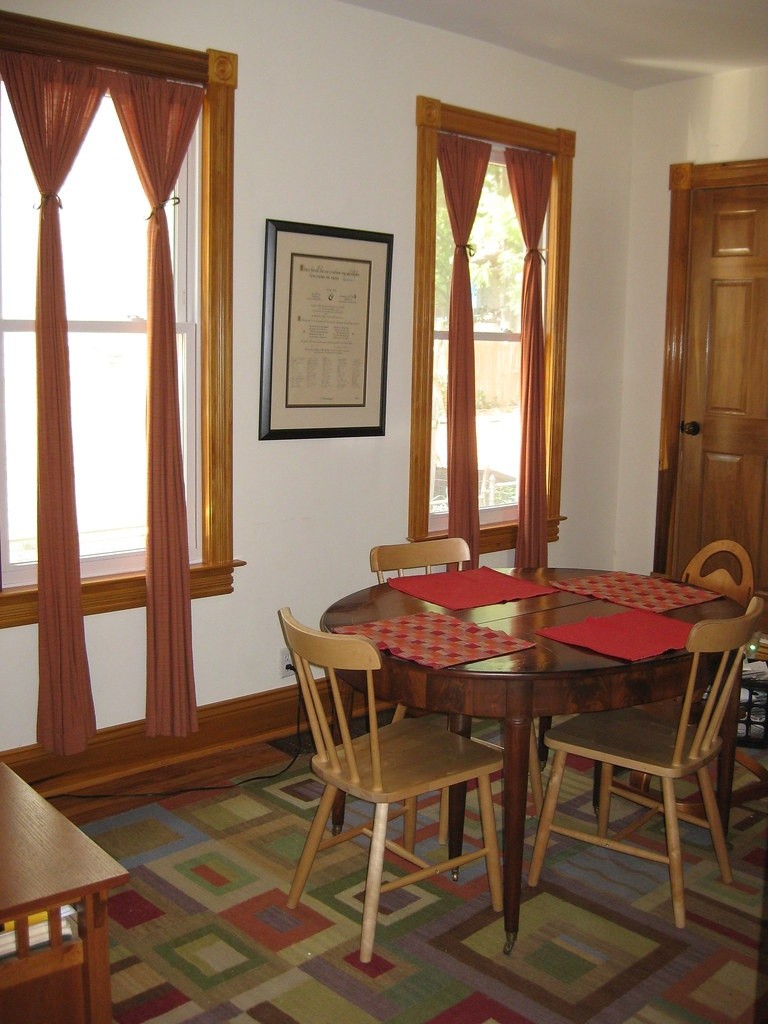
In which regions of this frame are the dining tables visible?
[318,567,745,953]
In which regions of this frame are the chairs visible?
[528,592,764,928]
[277,607,504,962]
[368,537,542,846]
[628,538,768,795]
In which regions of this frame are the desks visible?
[0,757,131,1024]
